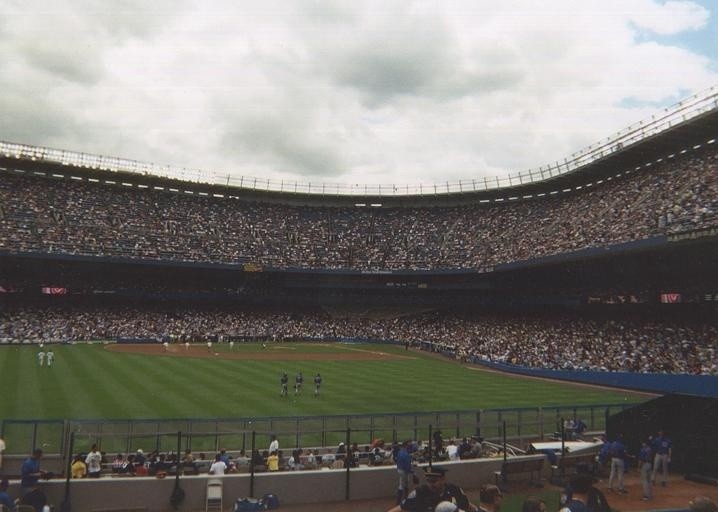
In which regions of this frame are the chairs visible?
[204,479,223,512]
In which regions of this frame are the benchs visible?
[491,459,547,485]
[550,451,601,484]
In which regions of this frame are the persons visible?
[280,372,288,398]
[262,343,266,349]
[47,349,55,367]
[574,416,587,437]
[1,171,410,338]
[562,416,573,437]
[161,342,168,351]
[412,146,716,375]
[687,494,717,510]
[403,340,410,350]
[37,350,46,366]
[314,372,321,396]
[185,342,191,350]
[228,341,233,351]
[294,371,304,397]
[1,428,675,509]
[207,340,213,352]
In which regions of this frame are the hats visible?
[422,466,448,480]
